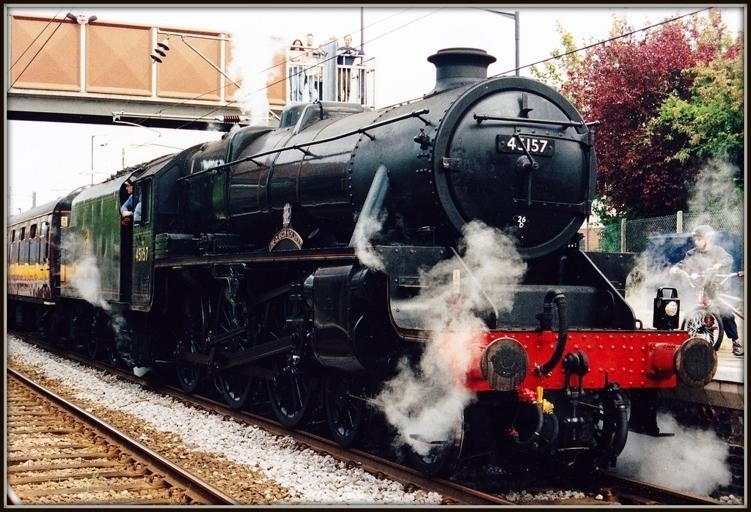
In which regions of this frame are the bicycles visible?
[676,266,745,352]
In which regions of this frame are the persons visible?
[120,176,137,218]
[678,224,744,357]
[289,32,365,103]
[132,187,142,223]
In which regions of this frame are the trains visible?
[7,44,719,496]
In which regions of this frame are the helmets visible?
[693,225,714,239]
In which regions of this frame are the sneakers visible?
[733,339,743,356]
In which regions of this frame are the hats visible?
[125,176,136,185]
